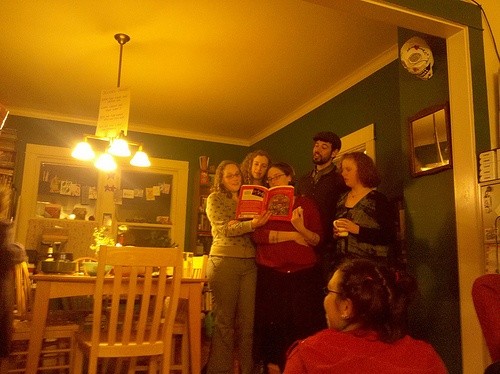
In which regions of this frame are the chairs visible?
[72,245,183,374]
[13,253,207,374]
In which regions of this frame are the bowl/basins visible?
[42,260,79,274]
[80,262,113,277]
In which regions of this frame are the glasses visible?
[321,286,343,296]
[266,174,286,183]
[222,172,241,179]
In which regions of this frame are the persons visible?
[240,150,272,184]
[283,258,448,374]
[297,131,341,280]
[332,152,397,261]
[250,161,323,374]
[206,161,271,374]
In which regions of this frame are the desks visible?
[26,275,208,374]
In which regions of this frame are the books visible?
[235,184,294,220]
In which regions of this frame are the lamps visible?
[70,33,152,171]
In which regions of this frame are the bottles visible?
[115,234,126,247]
[45,245,55,261]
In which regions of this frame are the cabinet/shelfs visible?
[191,169,217,256]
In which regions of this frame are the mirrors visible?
[407,102,454,178]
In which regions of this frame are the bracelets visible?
[272,230,278,243]
[305,232,316,245]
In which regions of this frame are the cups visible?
[333,220,349,237]
[182,252,194,279]
